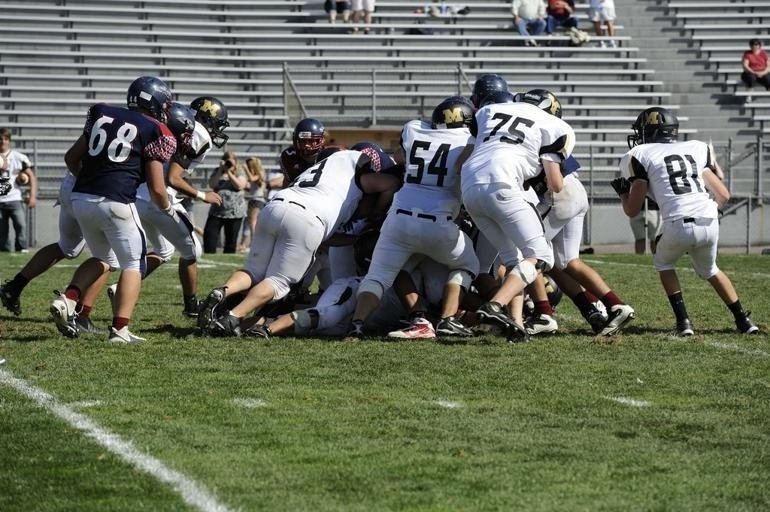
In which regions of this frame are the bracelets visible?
[161,201,172,215]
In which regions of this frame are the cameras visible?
[224,160,231,168]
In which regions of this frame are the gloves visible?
[610,176,630,195]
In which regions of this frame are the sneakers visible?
[387,294,558,345]
[0,280,22,316]
[342,319,368,339]
[182,286,272,338]
[341,217,374,236]
[582,299,636,336]
[676,318,694,336]
[50,283,147,344]
[734,310,759,335]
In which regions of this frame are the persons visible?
[0,103,195,337]
[589,0,617,48]
[510,0,547,46]
[324,0,352,34]
[351,0,375,34]
[611,108,759,334]
[741,39,770,103]
[50,77,180,344]
[108,97,229,316]
[0,128,36,253]
[546,0,579,46]
[619,196,662,255]
[196,74,634,340]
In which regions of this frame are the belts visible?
[274,198,324,225]
[396,209,452,222]
[683,218,695,223]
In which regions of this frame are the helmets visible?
[127,76,231,151]
[293,118,328,163]
[627,107,679,150]
[543,273,563,312]
[431,74,562,129]
